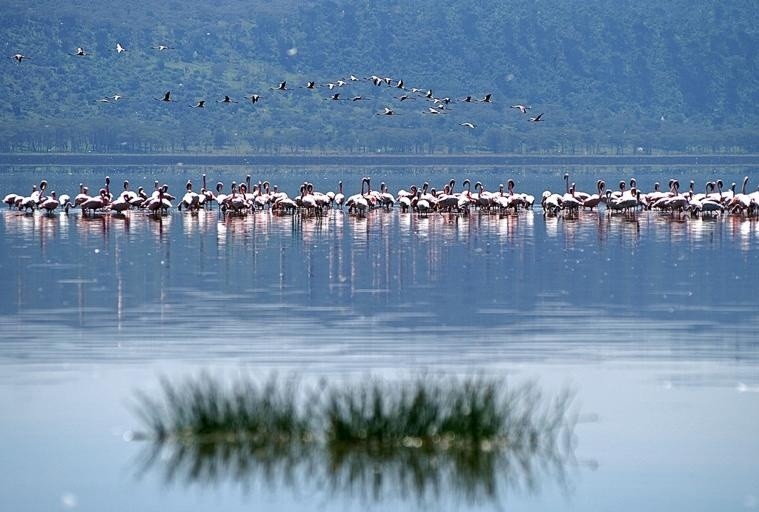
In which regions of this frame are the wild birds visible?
[108,43,127,54]
[510,103,532,113]
[150,46,175,52]
[456,94,494,104]
[397,178,535,215]
[365,75,410,91]
[458,122,478,129]
[380,94,413,116]
[94,99,114,104]
[527,113,545,122]
[68,48,94,58]
[6,175,176,214]
[325,93,371,102]
[189,94,265,108]
[269,76,363,91]
[104,95,122,102]
[6,54,32,62]
[412,87,453,115]
[153,91,180,103]
[540,174,759,218]
[346,178,394,214]
[177,174,344,214]
